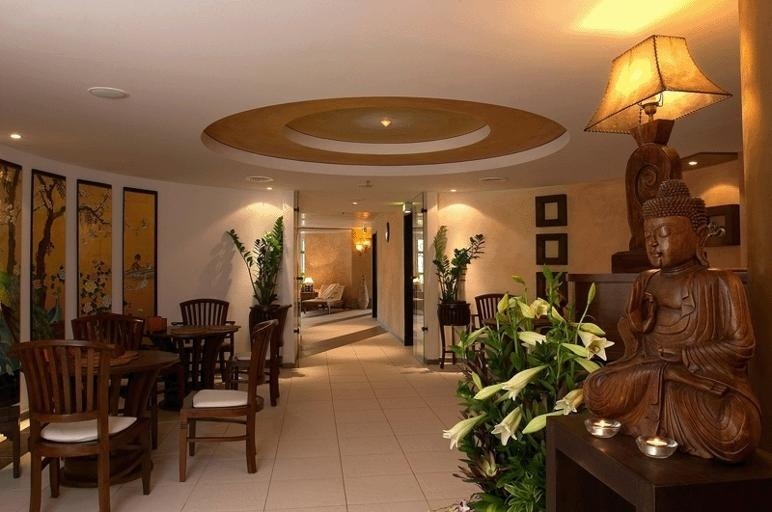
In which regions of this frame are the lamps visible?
[584,35,732,273]
[303,277,313,291]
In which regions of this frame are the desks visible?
[10,342,179,489]
[568,271,748,361]
[546,415,772,512]
[300,292,318,312]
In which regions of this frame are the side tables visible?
[439,323,470,369]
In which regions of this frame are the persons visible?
[583,178,762,464]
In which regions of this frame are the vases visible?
[358,272,369,309]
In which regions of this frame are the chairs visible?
[16,337,154,512]
[302,284,344,314]
[177,318,276,483]
[475,293,516,328]
[147,299,280,411]
[69,318,146,352]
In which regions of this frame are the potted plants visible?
[225,216,292,345]
[432,225,485,325]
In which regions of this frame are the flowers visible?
[442,265,615,512]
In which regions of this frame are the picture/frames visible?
[30,168,67,341]
[76,178,113,318]
[0,159,23,374]
[123,186,158,317]
